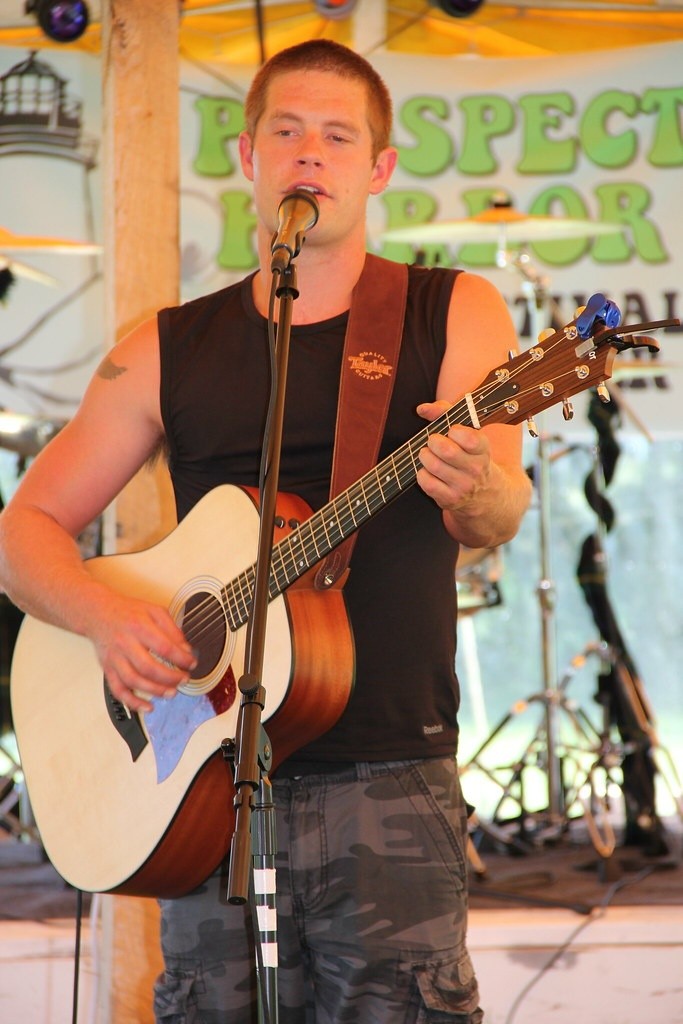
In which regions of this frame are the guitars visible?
[8,294,682,901]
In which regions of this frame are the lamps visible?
[24,0,88,42]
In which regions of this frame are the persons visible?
[0,38,532,1024]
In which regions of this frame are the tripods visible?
[457,434,683,881]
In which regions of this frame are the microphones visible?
[270,189,321,275]
